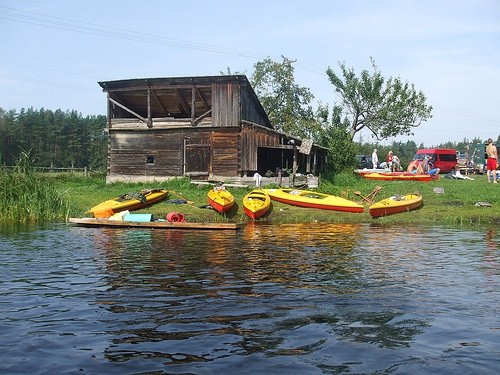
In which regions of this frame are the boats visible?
[368,193,423,217]
[207,181,235,214]
[68,217,246,230]
[85,188,170,217]
[352,167,433,182]
[242,188,273,219]
[262,188,370,214]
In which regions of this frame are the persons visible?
[387,151,400,172]
[484,137,499,184]
[372,149,378,170]
[252,170,263,188]
[450,165,477,181]
[408,156,440,176]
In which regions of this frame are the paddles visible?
[167,189,195,206]
[354,186,382,204]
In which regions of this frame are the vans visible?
[408,147,458,173]
